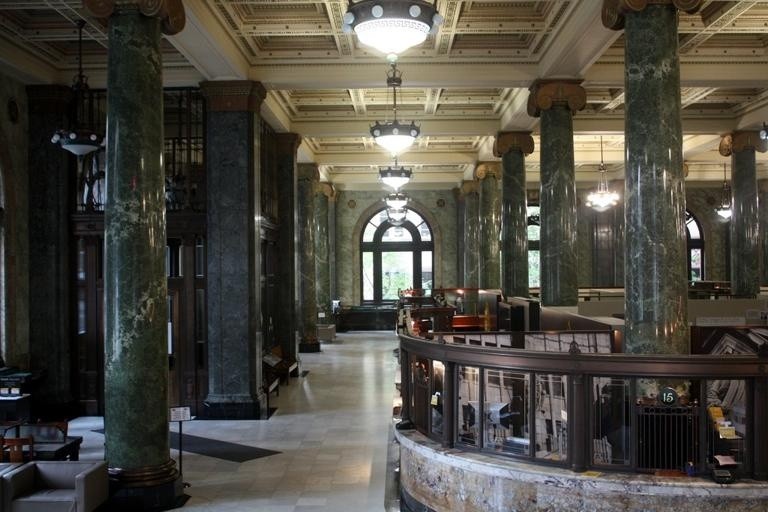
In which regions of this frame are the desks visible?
[0,387,32,420]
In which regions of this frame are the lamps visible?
[381,192,408,228]
[379,162,413,196]
[49,19,103,158]
[341,0,441,62]
[721,163,732,210]
[585,135,621,213]
[366,63,422,160]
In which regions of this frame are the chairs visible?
[0,420,83,460]
[1,462,107,512]
